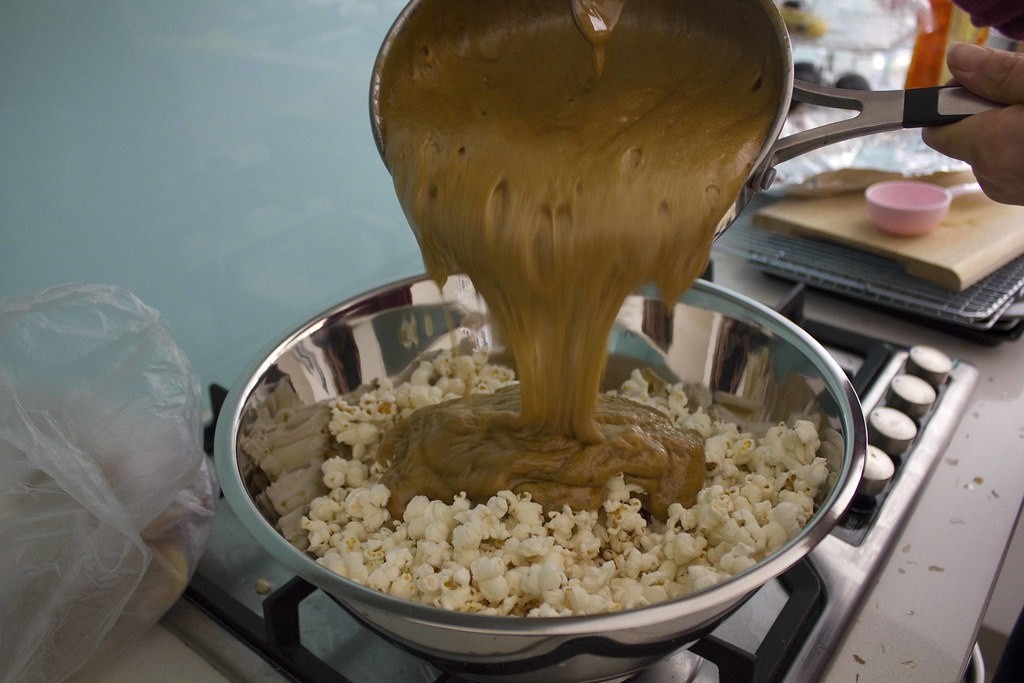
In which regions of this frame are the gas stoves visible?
[165,273,978,683]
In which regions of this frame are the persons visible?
[921,0,1024,207]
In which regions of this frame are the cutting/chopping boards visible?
[751,183,1024,290]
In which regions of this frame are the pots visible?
[372,1,1004,177]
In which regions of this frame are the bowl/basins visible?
[212,265,867,683]
[867,181,953,237]
